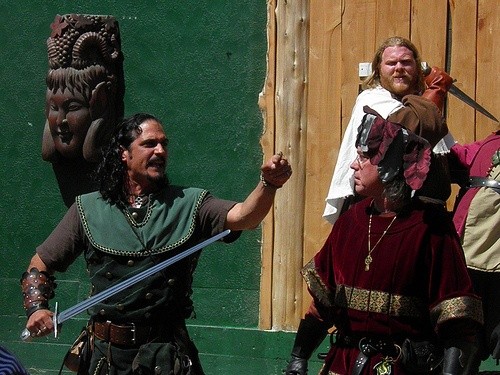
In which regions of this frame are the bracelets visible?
[260,173,282,190]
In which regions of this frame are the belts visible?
[335,330,431,357]
[88,321,171,346]
[465,178,500,189]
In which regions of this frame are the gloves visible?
[286,319,327,375]
[419,66,453,107]
[442,344,481,375]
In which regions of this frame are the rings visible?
[41,327,47,333]
[37,330,42,336]
[286,171,290,176]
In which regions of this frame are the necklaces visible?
[126,184,155,206]
[365,199,397,271]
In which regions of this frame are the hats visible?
[356,105,431,190]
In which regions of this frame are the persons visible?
[322,36,458,225]
[441,130,500,375]
[42,13,125,162]
[286,104,484,375]
[22,114,292,375]
